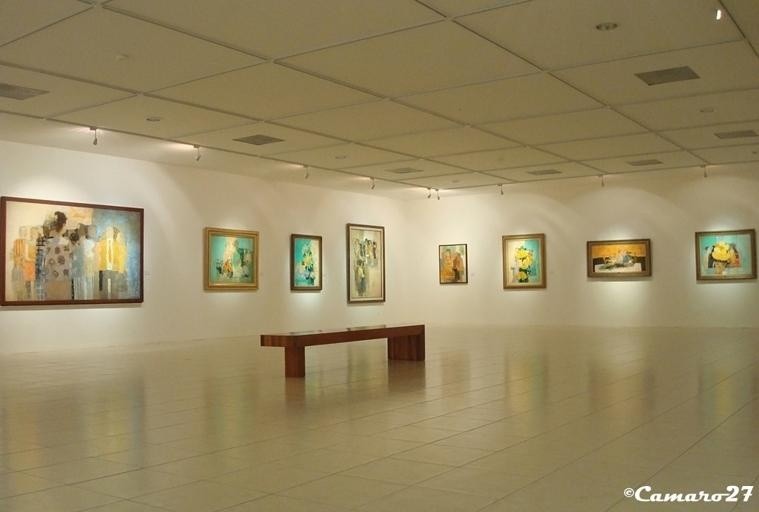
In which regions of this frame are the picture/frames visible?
[500,229,757,290]
[438,243,469,285]
[202,223,387,305]
[1,195,146,306]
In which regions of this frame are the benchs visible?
[259,321,427,378]
